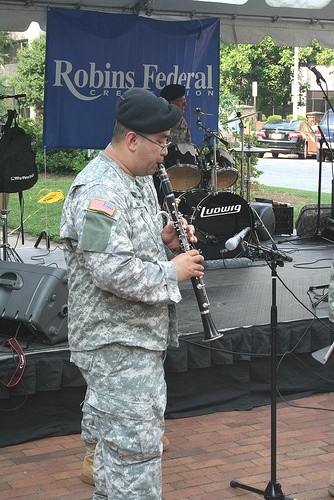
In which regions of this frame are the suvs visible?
[314,108,334,163]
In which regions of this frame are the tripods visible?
[277,140,333,244]
[0,94,26,263]
[230,236,297,500]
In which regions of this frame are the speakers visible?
[0,259,69,346]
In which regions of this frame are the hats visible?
[115,87,182,133]
[160,84,185,101]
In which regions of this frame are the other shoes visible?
[162,437,169,451]
[81,456,94,486]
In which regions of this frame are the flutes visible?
[158,163,225,343]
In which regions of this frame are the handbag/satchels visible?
[0,109,38,193]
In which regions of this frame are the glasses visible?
[125,130,172,152]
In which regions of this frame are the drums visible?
[200,146,238,189]
[176,189,253,260]
[163,142,202,189]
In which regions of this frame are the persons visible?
[59,88,205,500]
[160,84,191,145]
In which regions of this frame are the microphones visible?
[308,65,326,83]
[225,227,252,251]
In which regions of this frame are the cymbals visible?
[223,112,256,124]
[229,147,271,153]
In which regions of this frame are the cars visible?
[256,119,316,159]
